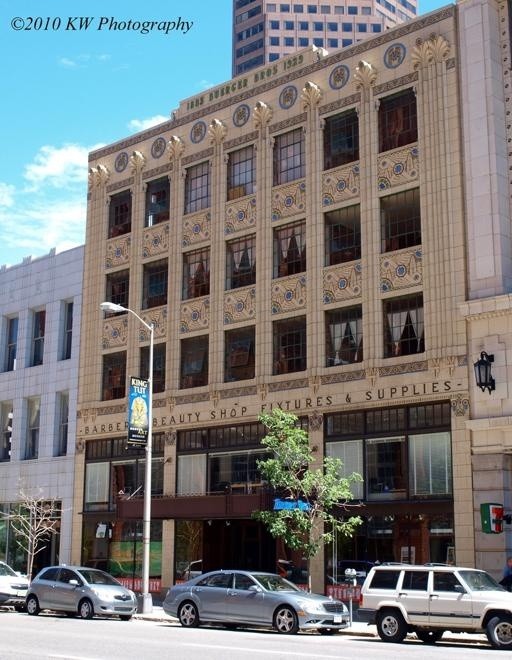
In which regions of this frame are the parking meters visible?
[344,568,357,626]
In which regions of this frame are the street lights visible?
[100,302,154,614]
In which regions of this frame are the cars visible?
[84,558,142,579]
[327,560,374,582]
[0,560,138,621]
[164,569,350,633]
[182,560,203,580]
[282,570,338,585]
[358,562,512,649]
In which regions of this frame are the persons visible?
[502,556,511,577]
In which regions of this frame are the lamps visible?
[473,352,494,394]
